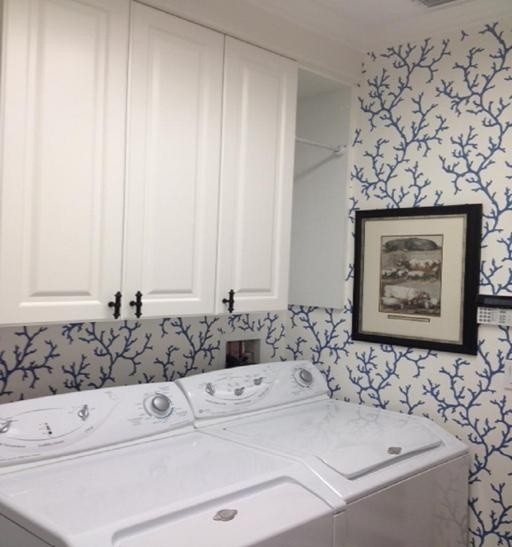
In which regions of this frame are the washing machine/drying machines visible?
[0,381,347,547]
[176,360,470,546]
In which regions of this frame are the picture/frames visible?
[351,203,483,356]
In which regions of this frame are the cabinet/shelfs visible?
[214,33,299,315]
[0,0,225,327]
[288,62,354,310]
